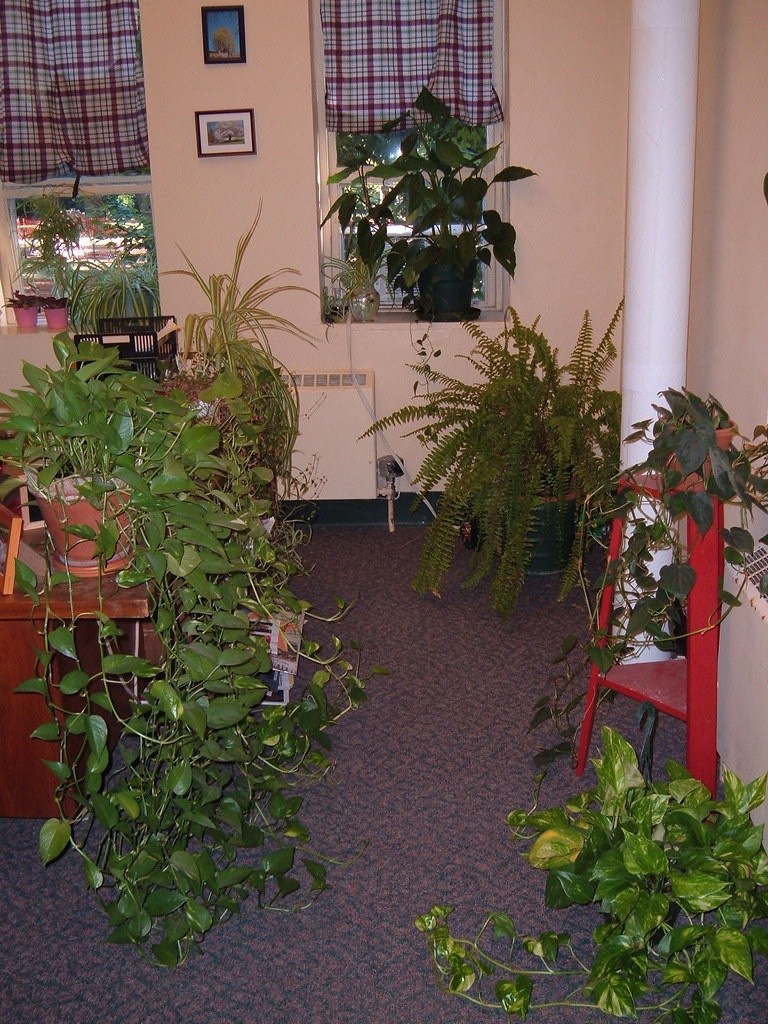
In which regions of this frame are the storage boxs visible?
[72,315,178,382]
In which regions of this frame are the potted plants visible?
[0,84,768,1024]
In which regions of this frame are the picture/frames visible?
[194,108,257,158]
[201,5,246,64]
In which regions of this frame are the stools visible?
[575,476,724,800]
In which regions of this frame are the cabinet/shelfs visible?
[0,528,163,819]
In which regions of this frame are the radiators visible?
[273,368,378,501]
